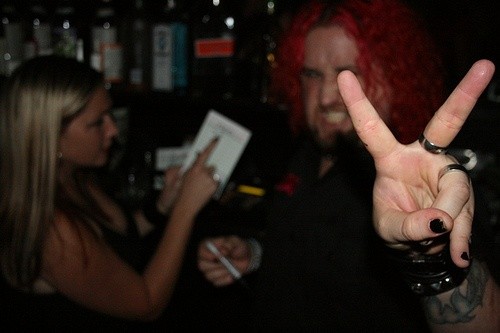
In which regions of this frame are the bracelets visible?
[143,204,169,226]
[403,244,471,296]
[248,236,261,273]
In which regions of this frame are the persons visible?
[0,55,220,333]
[337,60,500,333]
[197,0,500,333]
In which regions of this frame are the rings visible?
[439,164,471,182]
[419,132,449,155]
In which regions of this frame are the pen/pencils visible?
[205,243,242,279]
